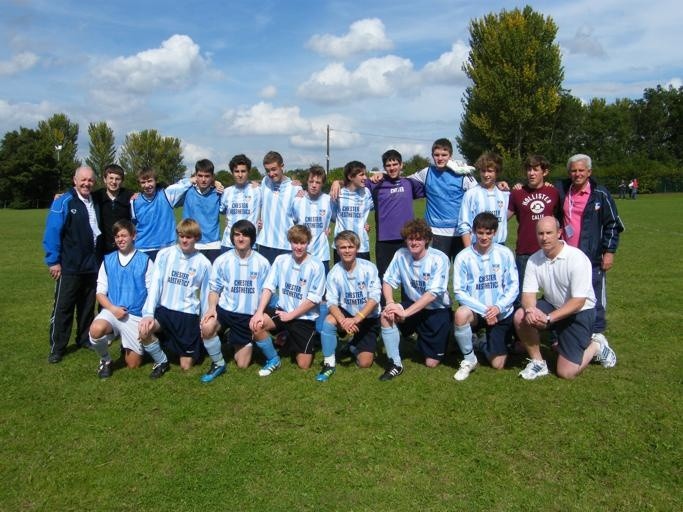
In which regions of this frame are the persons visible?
[43,137,626,364]
[512,217,616,380]
[374,218,451,381]
[630,178,638,200]
[86,219,154,378]
[247,224,326,377]
[198,218,271,383]
[315,229,384,381]
[627,179,634,199]
[451,210,521,379]
[136,217,214,379]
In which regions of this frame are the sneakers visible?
[85,344,94,352]
[519,358,546,380]
[200,362,226,380]
[592,333,616,368]
[97,360,113,378]
[49,354,62,363]
[473,334,478,345]
[454,357,478,381]
[108,333,117,346]
[315,362,336,380]
[476,334,487,350]
[150,359,170,379]
[258,359,282,377]
[275,330,288,346]
[381,363,403,380]
